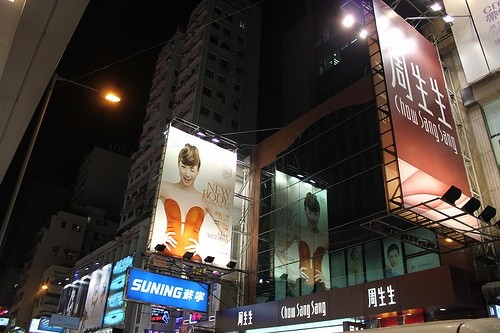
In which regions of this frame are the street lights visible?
[0,72,121,248]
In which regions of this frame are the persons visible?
[66,289,76,317]
[157,142,232,264]
[275,192,330,288]
[350,246,360,286]
[385,243,403,278]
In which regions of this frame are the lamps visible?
[440,184,500,230]
